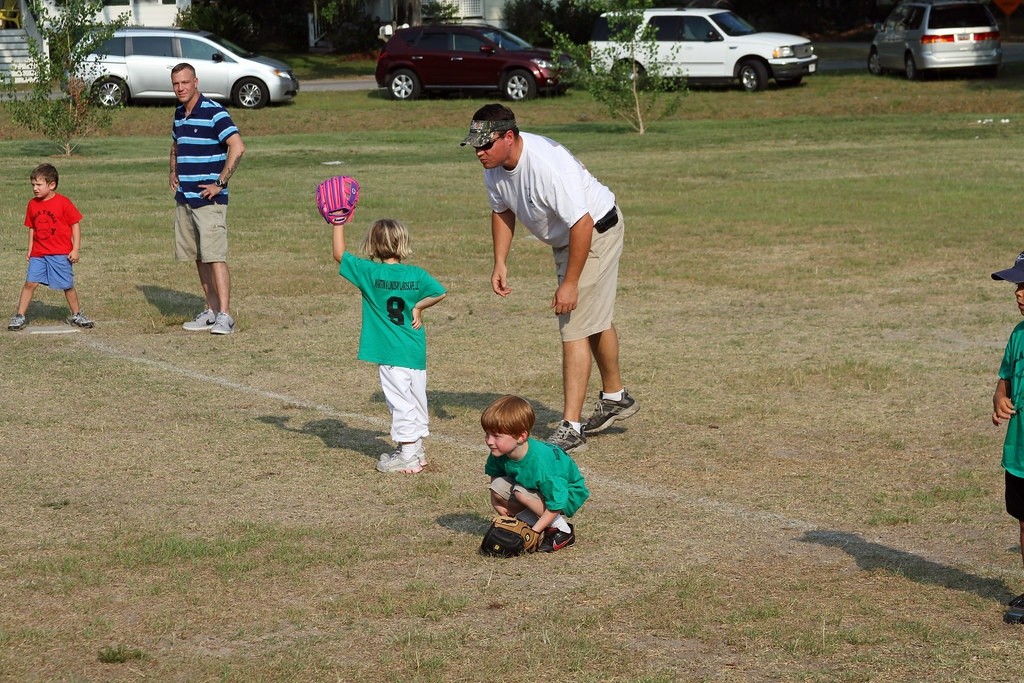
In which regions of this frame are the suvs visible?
[867,0,1004,81]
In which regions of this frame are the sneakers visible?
[376,445,428,474]
[182,309,216,330]
[536,523,575,551]
[584,387,640,432]
[7,313,26,331]
[210,311,235,335]
[66,312,94,328]
[542,420,589,454]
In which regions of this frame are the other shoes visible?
[1003,593,1024,624]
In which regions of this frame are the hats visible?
[460,120,516,146]
[991,250,1024,283]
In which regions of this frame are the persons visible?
[480,394,590,552]
[315,176,448,474]
[987,252,1024,623]
[8,163,95,330]
[169,63,245,335]
[461,104,640,450]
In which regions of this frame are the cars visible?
[586,7,818,93]
[58,25,300,109]
[375,23,581,101]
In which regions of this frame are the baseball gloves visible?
[480,516,545,559]
[315,175,361,225]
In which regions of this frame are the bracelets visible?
[170,171,176,173]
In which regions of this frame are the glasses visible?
[474,130,509,150]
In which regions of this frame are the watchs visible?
[215,178,228,189]
